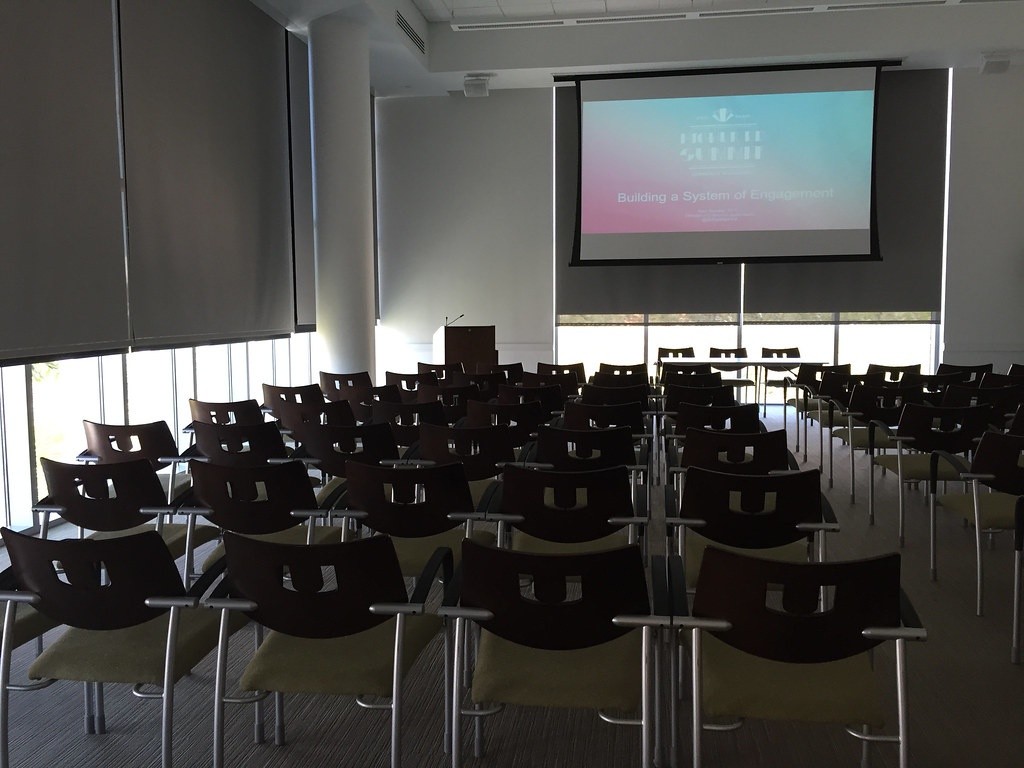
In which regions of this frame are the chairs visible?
[670,544,929,768]
[204,529,469,768]
[1,526,266,768]
[1,346,1024,682]
[438,537,668,768]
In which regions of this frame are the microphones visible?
[445,313,464,326]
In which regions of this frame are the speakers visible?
[463,80,489,97]
[979,56,1011,74]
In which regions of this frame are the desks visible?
[659,356,830,417]
